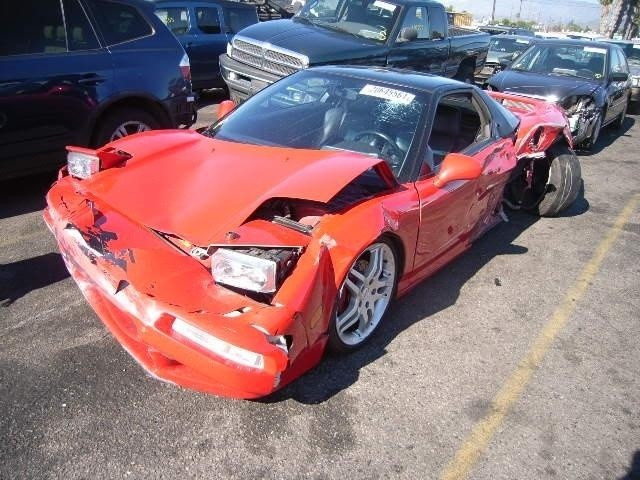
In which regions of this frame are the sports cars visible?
[30,59,589,404]
